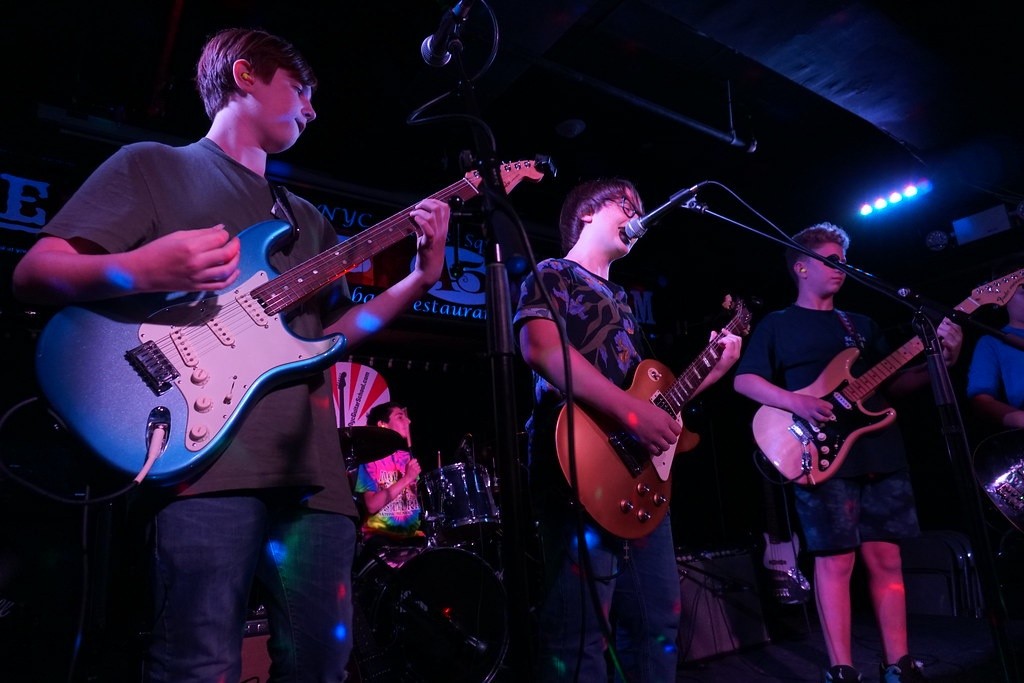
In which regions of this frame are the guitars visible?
[972,426,1024,535]
[547,293,754,541]
[761,484,812,606]
[31,157,559,494]
[751,268,1024,488]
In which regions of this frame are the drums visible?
[416,459,503,545]
[350,544,512,683]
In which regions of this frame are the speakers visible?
[674,547,772,663]
[238,617,272,683]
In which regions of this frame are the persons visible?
[515,177,742,683]
[731,221,963,683]
[967,262,1024,429]
[355,403,421,537]
[15,30,450,683]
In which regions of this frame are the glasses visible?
[601,195,643,221]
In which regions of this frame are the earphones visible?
[800,268,806,273]
[242,73,254,85]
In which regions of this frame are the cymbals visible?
[337,425,408,472]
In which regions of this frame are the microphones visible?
[421,0,473,68]
[625,180,708,239]
[464,639,488,651]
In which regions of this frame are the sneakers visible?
[879,654,928,683]
[824,663,863,683]
